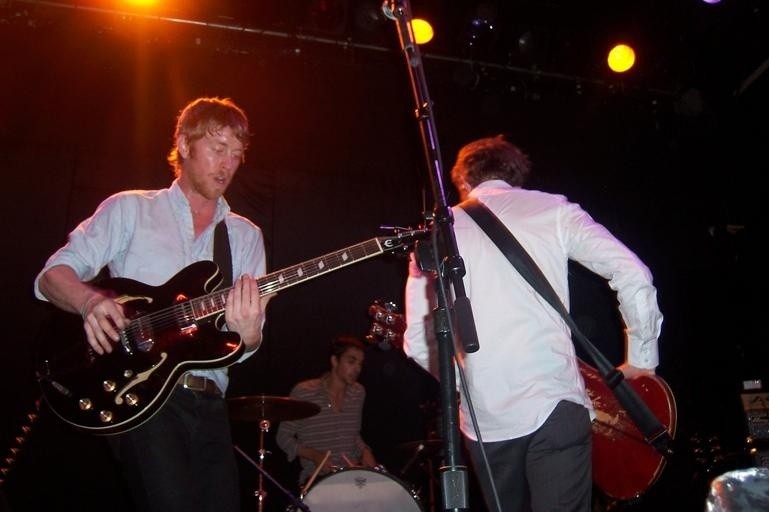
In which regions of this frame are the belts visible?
[176,373,222,397]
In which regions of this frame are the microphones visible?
[382,0,400,19]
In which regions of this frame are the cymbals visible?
[390,438,449,459]
[226,396,319,421]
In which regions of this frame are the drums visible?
[287,465,425,511]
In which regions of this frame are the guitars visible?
[35,226,430,437]
[363,300,677,500]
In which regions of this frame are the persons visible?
[403,138,663,511]
[276,336,376,490]
[34,97,276,398]
